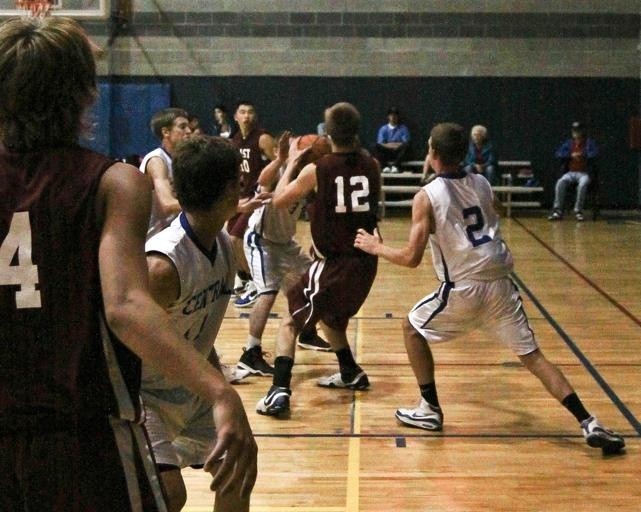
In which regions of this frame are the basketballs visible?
[299,135,332,162]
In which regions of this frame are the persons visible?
[352,122,626,450]
[373,103,413,172]
[547,120,601,220]
[461,124,498,186]
[0,12,258,511]
[316,102,336,135]
[213,103,240,138]
[139,106,194,242]
[235,127,334,377]
[189,115,204,136]
[224,99,278,308]
[138,134,251,512]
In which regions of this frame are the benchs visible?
[378,159,545,218]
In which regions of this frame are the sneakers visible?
[221,326,370,415]
[233,280,261,308]
[391,162,400,173]
[395,396,443,431]
[548,209,563,220]
[574,212,585,221]
[580,414,625,449]
[382,164,391,172]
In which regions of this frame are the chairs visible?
[551,156,600,222]
